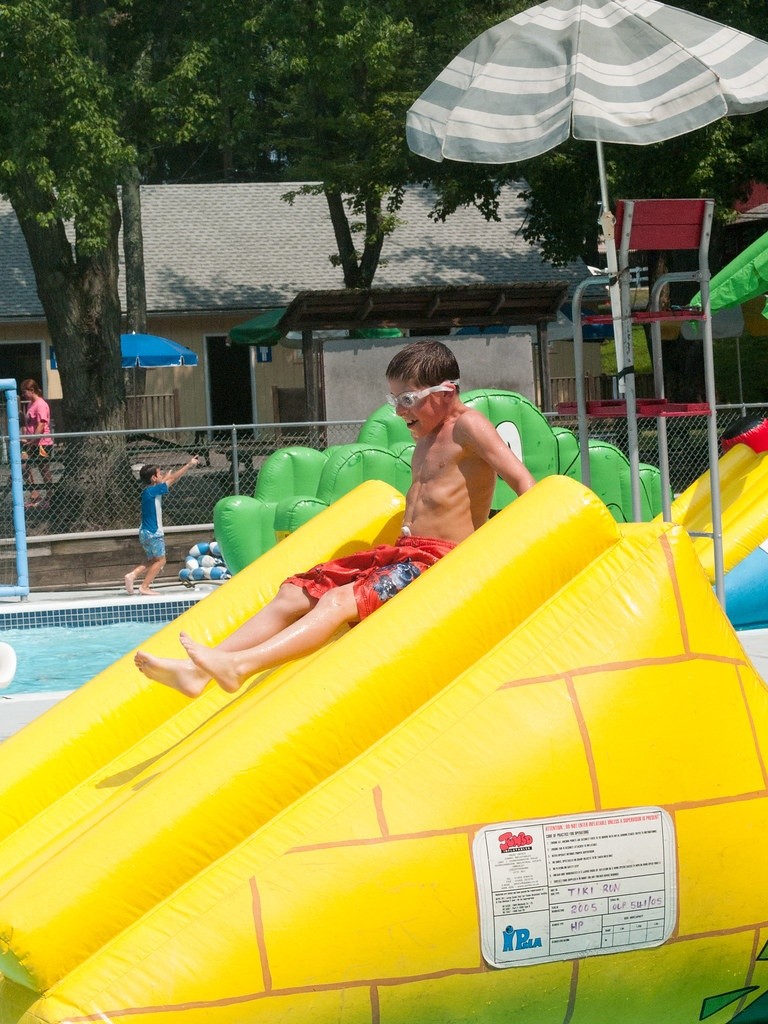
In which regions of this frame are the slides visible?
[0,388,768,1024]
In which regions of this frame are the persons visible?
[18,379,54,501]
[125,455,200,595]
[133,340,535,698]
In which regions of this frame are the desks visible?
[0,456,66,506]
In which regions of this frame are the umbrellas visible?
[120,334,199,366]
[405,0,768,393]
[229,308,404,348]
[690,231,768,320]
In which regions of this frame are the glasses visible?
[385,385,454,408]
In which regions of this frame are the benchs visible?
[127,445,213,467]
[0,483,61,491]
[215,446,277,472]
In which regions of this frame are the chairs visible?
[573,197,725,610]
